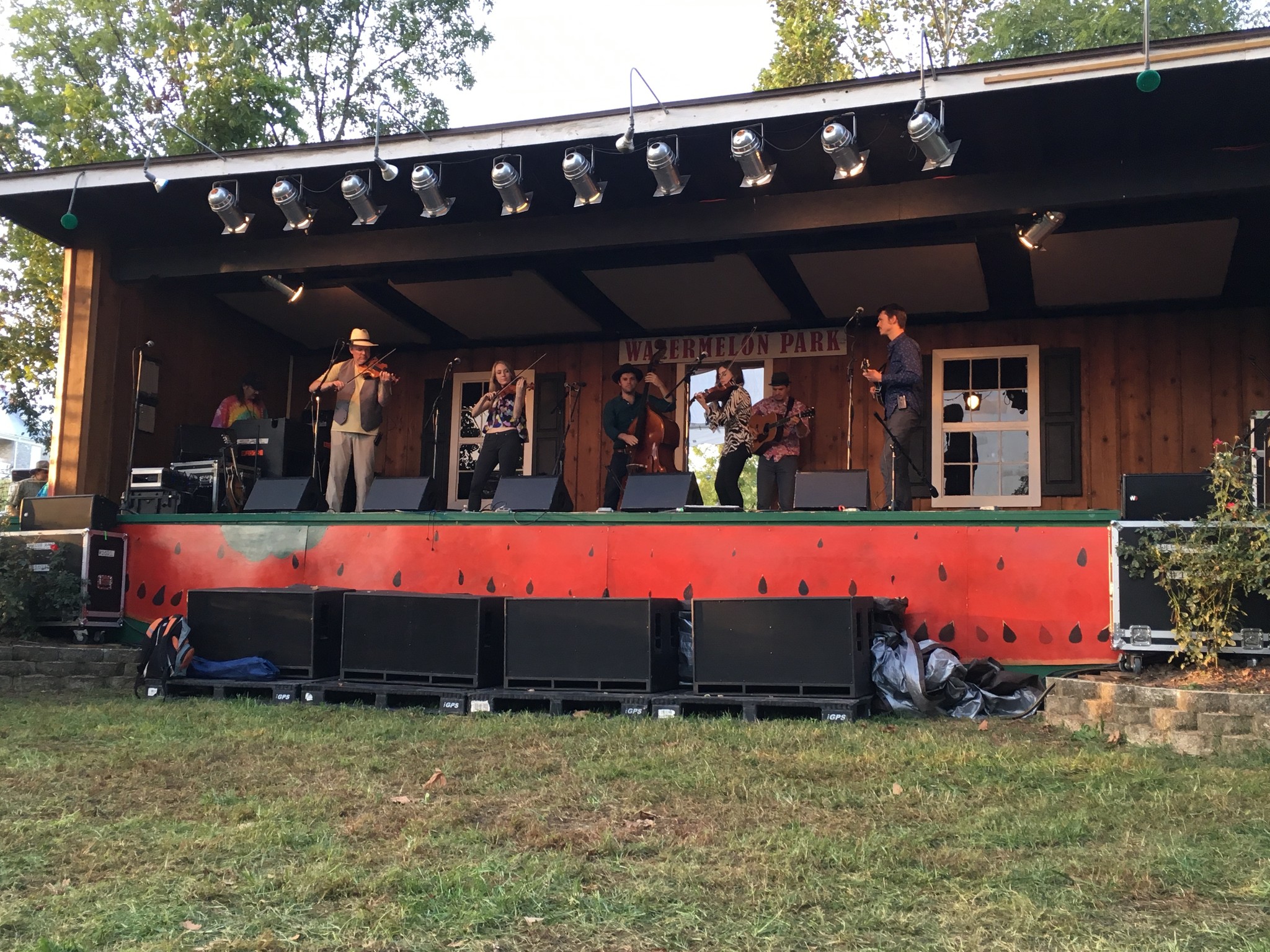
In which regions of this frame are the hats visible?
[342,328,378,346]
[768,371,791,386]
[29,460,49,474]
[611,363,643,385]
[248,369,268,389]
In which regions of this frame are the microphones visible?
[134,341,155,349]
[695,352,709,361]
[339,337,353,345]
[855,307,864,315]
[573,382,587,387]
[928,484,939,498]
[449,358,461,366]
[564,383,578,392]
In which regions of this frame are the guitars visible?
[222,433,248,513]
[749,407,815,457]
[861,358,885,407]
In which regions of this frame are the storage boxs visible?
[170,460,261,513]
[1110,520,1270,654]
[0,527,129,627]
[1242,410,1270,519]
[188,586,876,699]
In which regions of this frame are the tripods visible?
[119,348,144,516]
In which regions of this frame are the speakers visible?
[1120,473,1250,521]
[243,476,329,513]
[362,475,448,511]
[174,423,239,463]
[793,470,872,510]
[187,587,895,697]
[490,475,575,513]
[620,471,704,513]
[19,494,118,531]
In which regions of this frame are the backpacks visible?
[137,613,194,680]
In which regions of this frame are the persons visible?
[749,372,812,511]
[602,363,676,511]
[4,460,50,516]
[309,328,393,512]
[694,360,754,512]
[211,373,268,428]
[468,360,526,512]
[863,303,924,511]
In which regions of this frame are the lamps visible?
[374,101,431,181]
[962,391,982,411]
[561,143,608,208]
[729,122,777,189]
[907,28,962,173]
[339,169,388,225]
[411,161,456,219]
[207,179,256,235]
[820,109,872,181]
[271,174,318,235]
[259,272,305,303]
[143,121,227,193]
[1014,210,1066,251]
[492,154,534,216]
[646,133,691,197]
[615,67,669,154]
[1003,388,1027,414]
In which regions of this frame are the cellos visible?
[621,339,682,490]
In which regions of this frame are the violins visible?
[689,381,739,406]
[499,377,535,398]
[358,357,400,385]
